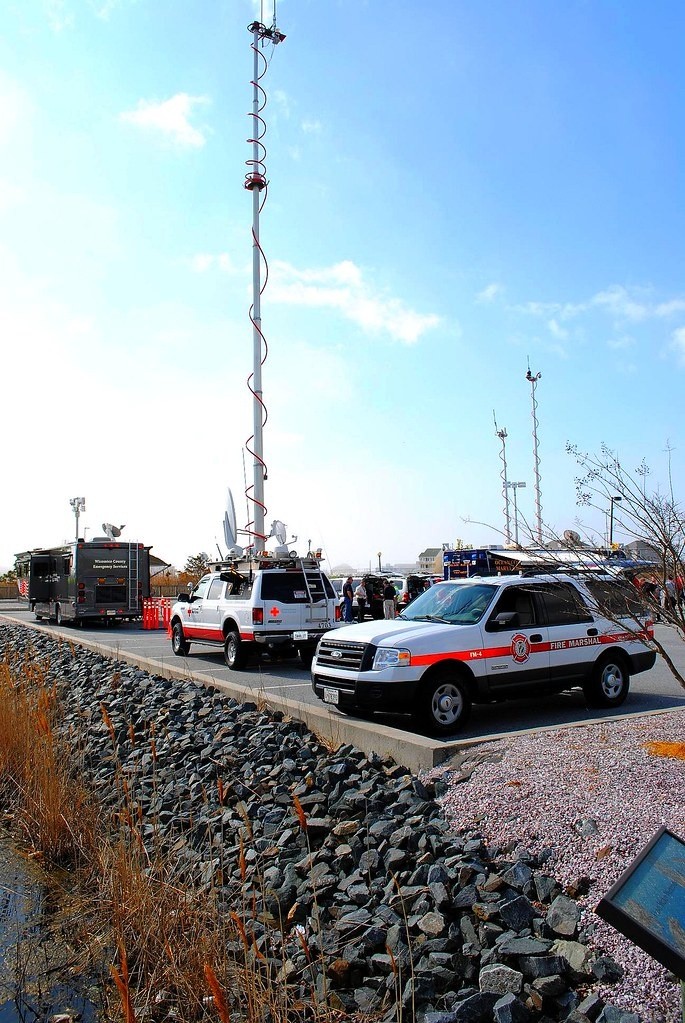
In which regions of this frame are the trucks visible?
[14,536,171,626]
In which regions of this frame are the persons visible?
[343,577,354,624]
[390,581,400,604]
[641,575,685,622]
[421,582,429,592]
[356,581,366,623]
[382,580,394,620]
[435,579,441,583]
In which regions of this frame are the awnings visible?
[489,550,660,570]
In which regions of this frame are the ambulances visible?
[169,547,341,673]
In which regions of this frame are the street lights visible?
[378,552,382,572]
[609,496,623,546]
[83,527,90,541]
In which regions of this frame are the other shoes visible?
[656,619,664,623]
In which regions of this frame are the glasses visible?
[350,580,353,581]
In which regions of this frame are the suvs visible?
[309,567,656,737]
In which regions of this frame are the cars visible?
[328,574,373,607]
[388,577,434,604]
